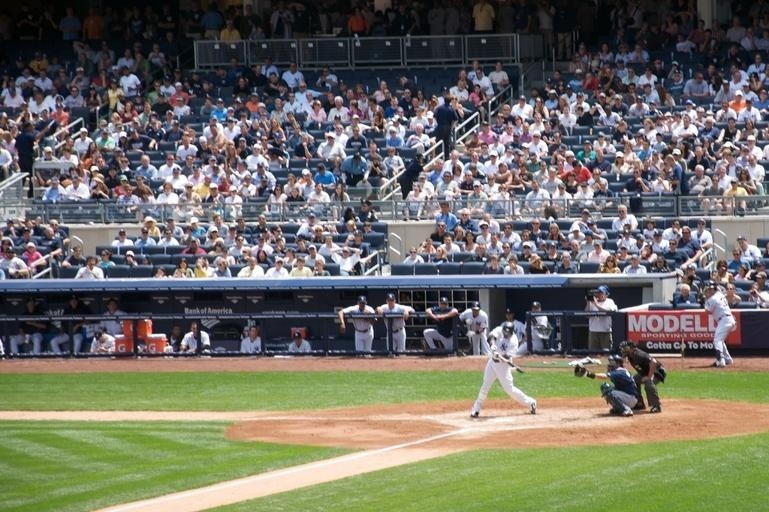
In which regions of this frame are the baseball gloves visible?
[574,364,588,376]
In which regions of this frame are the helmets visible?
[607,354,624,369]
[618,340,634,358]
[503,322,515,337]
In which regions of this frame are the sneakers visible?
[713,358,734,367]
[531,403,536,414]
[470,410,479,418]
[650,405,662,412]
[621,411,634,416]
[633,401,645,410]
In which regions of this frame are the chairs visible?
[1,46,767,277]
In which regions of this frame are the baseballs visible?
[511,367,516,372]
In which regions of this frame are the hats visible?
[472,300,480,311]
[118,216,269,245]
[94,328,104,341]
[504,306,512,314]
[356,295,368,305]
[531,301,543,308]
[440,297,448,305]
[385,292,396,302]
[546,208,596,253]
[597,285,610,297]
[154,71,272,186]
[581,92,694,163]
[292,332,302,340]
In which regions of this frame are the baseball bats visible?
[500,355,525,374]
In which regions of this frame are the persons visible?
[617,338,669,414]
[470,320,536,424]
[574,354,639,416]
